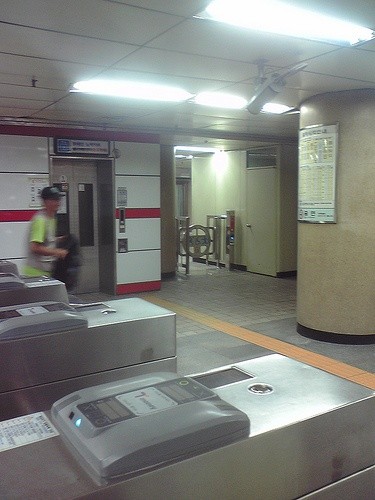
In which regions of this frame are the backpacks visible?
[53,233,82,291]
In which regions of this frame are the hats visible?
[41,186,65,200]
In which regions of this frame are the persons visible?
[24,185,70,278]
